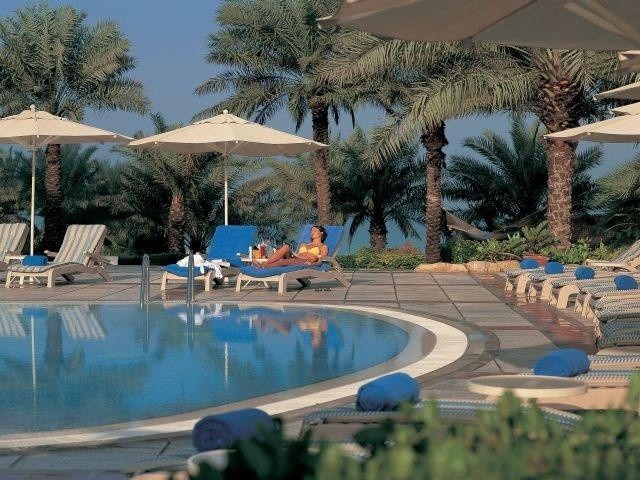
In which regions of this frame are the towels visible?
[189,408,278,451]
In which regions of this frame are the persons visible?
[256,226,330,269]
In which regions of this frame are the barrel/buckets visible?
[522,253,550,268]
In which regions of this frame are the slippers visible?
[315,287,324,290]
[324,287,331,291]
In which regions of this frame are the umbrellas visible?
[0,104,137,285]
[118,109,331,282]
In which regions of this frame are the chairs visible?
[160,221,261,291]
[164,306,347,352]
[0,217,28,285]
[130,450,196,480]
[3,220,115,289]
[504,241,639,348]
[1,304,27,343]
[236,221,350,296]
[299,347,638,462]
[8,304,109,342]
